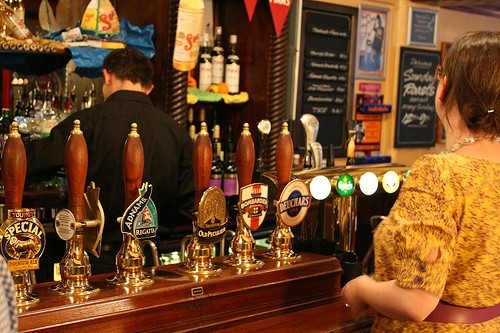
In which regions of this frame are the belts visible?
[424,301,500,324]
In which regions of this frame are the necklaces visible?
[438,136,487,155]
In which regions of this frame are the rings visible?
[345,303,350,307]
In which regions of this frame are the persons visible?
[341,30,500,333]
[25,48,195,283]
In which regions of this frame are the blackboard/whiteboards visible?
[395,46,443,149]
[297,10,351,150]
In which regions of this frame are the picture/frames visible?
[407,6,439,46]
[355,4,390,80]
[439,42,453,141]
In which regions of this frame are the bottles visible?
[187,107,239,210]
[0,83,97,191]
[194,25,240,95]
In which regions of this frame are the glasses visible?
[434,64,445,81]
